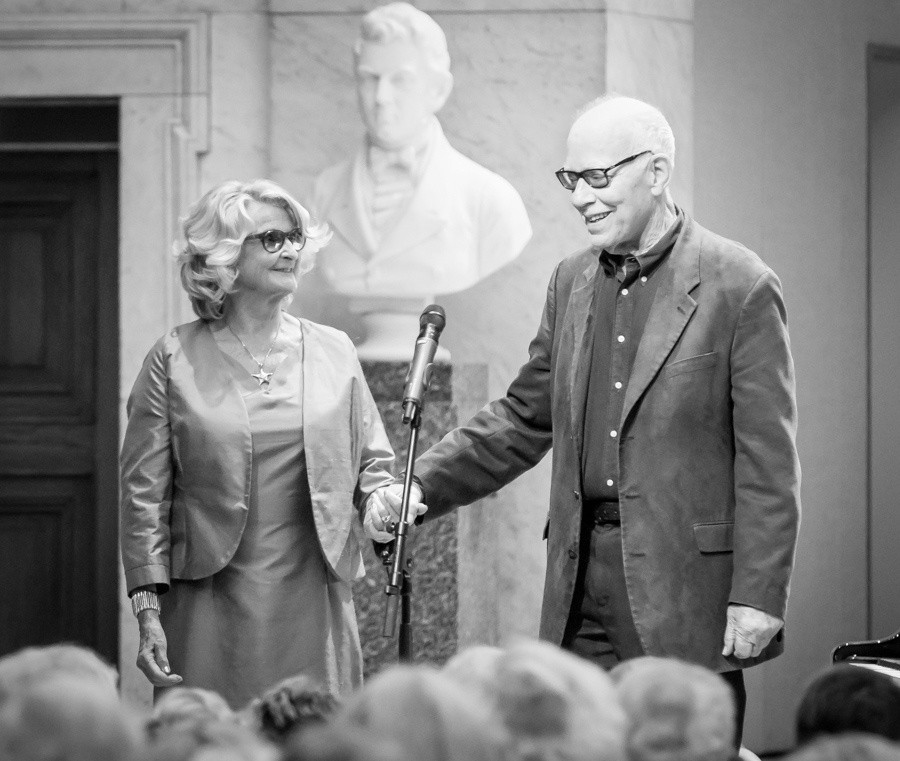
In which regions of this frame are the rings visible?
[382,514,391,523]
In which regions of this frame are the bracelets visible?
[131,591,161,617]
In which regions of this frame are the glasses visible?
[555,150,653,190]
[246,228,305,253]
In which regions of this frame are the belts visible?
[584,500,621,525]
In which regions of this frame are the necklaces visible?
[225,313,282,386]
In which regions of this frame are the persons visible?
[303,2,532,300]
[0,642,900,761]
[119,175,428,712]
[365,94,803,756]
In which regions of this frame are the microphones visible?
[401,305,446,424]
